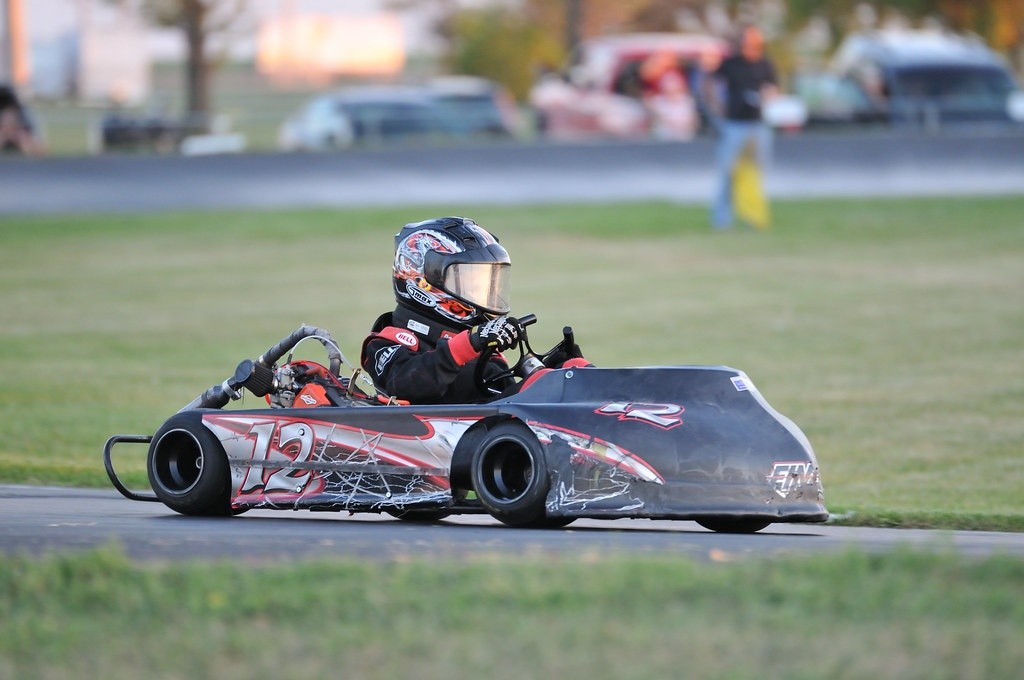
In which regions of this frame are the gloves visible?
[542,345,585,370]
[469,316,526,353]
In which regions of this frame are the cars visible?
[280,88,452,145]
[423,79,510,141]
[527,31,805,139]
[803,35,1022,130]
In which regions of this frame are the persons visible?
[708,22,780,233]
[0,84,47,156]
[826,3,897,128]
[530,52,723,142]
[362,218,582,405]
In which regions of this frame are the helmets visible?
[393,216,511,331]
